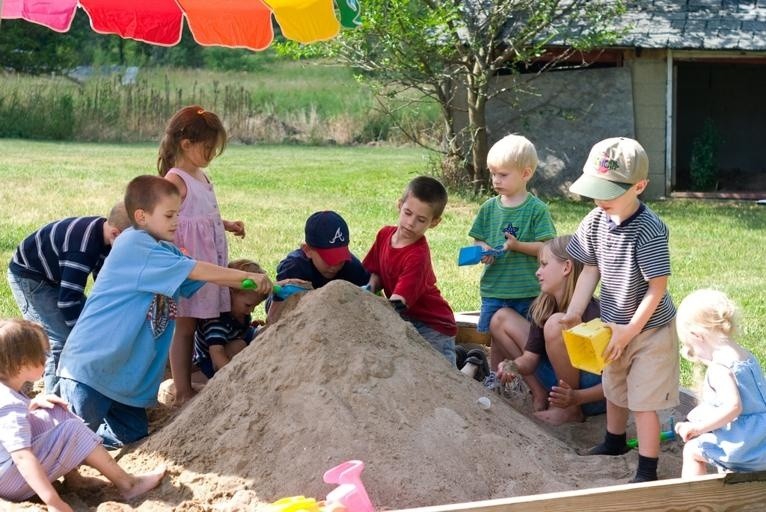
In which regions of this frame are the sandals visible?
[463,349,491,381]
[454,343,469,370]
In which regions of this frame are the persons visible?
[558,136,681,482]
[1,318,167,512]
[488,235,606,427]
[265,210,371,315]
[674,288,766,478]
[361,176,490,382]
[157,105,246,408]
[7,201,132,393]
[190,258,270,379]
[468,134,557,373]
[55,175,308,451]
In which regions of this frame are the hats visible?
[305,210,353,267]
[569,136,651,201]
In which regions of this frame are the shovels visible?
[458,245,509,266]
[241,279,309,300]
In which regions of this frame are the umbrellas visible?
[0,0,363,51]
